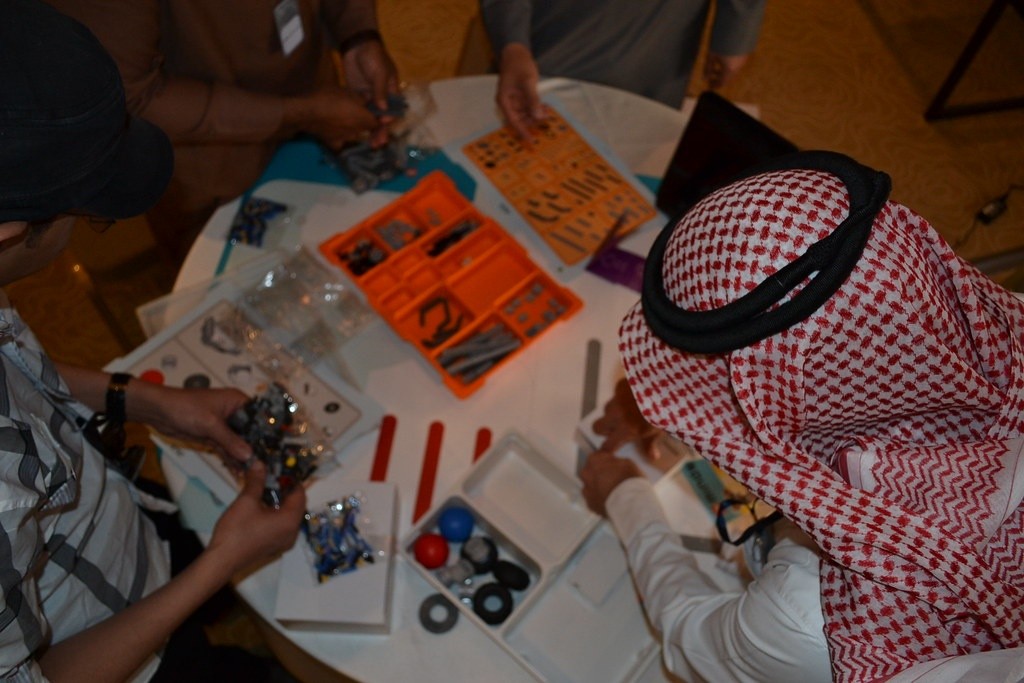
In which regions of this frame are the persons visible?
[0,1,770,236]
[0,304,308,683]
[576,146,1024,683]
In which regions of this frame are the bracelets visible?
[335,28,386,58]
[105,371,134,428]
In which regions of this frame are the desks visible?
[156,73,755,683]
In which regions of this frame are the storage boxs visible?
[398,433,662,683]
[318,169,584,401]
